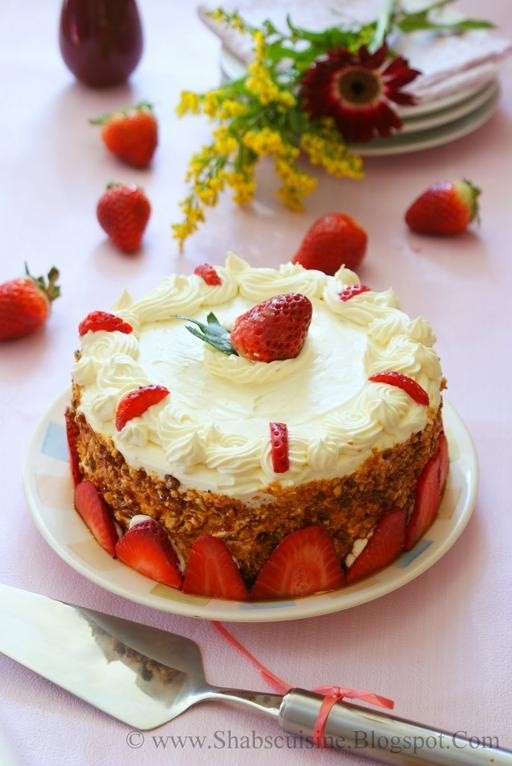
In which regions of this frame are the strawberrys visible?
[292,213,367,276]
[0,261,60,342]
[97,180,150,253]
[87,100,157,168]
[404,177,481,235]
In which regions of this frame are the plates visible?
[214,3,507,165]
[17,386,482,632]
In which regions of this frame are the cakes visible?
[63,250,449,603]
